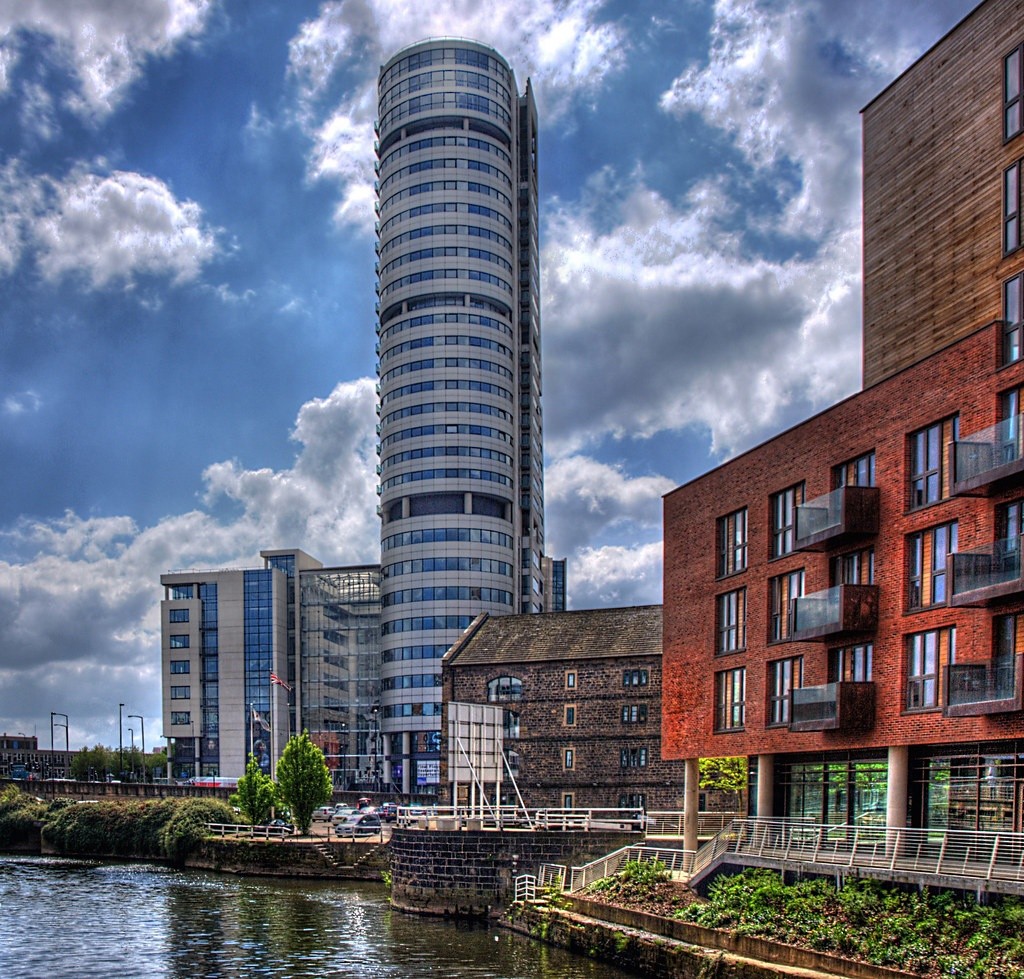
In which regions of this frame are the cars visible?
[311,797,429,841]
[255,818,295,835]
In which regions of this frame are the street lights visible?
[54,723,69,779]
[119,703,125,782]
[128,715,147,784]
[18,733,27,763]
[51,712,70,780]
[128,729,135,783]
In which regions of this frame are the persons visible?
[187,780,195,786]
[984,774,996,798]
[175,779,178,785]
